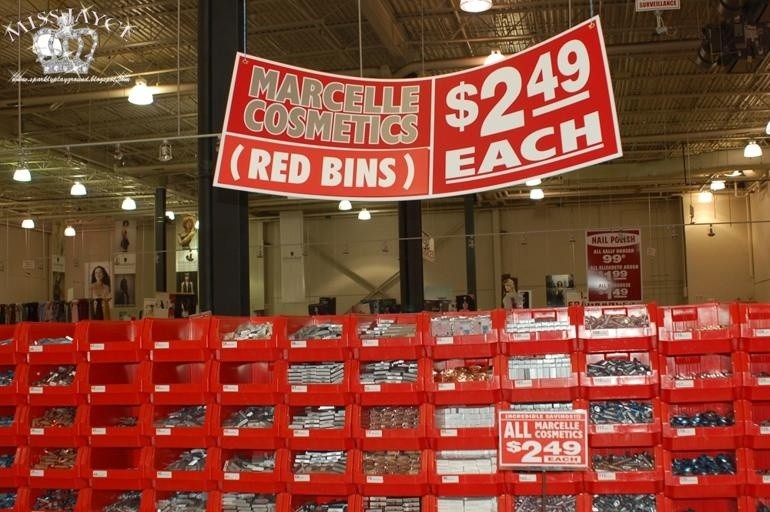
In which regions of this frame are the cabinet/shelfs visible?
[1,297,769,512]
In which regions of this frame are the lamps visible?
[0,0,770,239]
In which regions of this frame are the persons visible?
[178,217,196,251]
[181,273,196,294]
[502,278,520,308]
[556,280,565,303]
[119,220,131,250]
[89,265,110,298]
[459,296,471,311]
[119,274,129,305]
